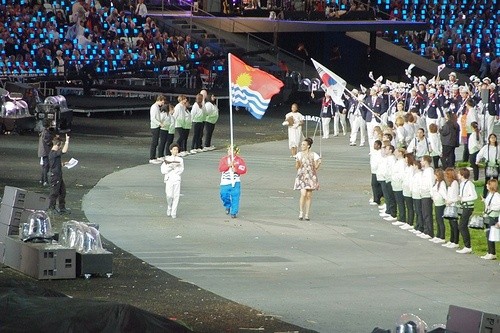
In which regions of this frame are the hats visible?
[351,88,358,95]
[427,87,436,95]
[418,75,427,82]
[370,85,379,93]
[482,77,491,83]
[448,71,457,78]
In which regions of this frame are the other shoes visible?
[39,180,50,187]
[50,208,71,215]
[466,167,473,170]
[334,134,339,136]
[369,198,397,220]
[456,247,472,254]
[441,241,460,248]
[290,155,296,158]
[321,136,330,139]
[392,220,414,230]
[429,237,445,243]
[225,209,237,218]
[298,214,310,221]
[51,137,63,142]
[481,251,497,260]
[349,142,365,148]
[167,209,177,219]
[407,229,432,239]
[343,132,347,136]
[149,145,216,164]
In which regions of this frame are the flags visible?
[438,64,445,72]
[369,71,374,79]
[230,54,284,119]
[312,60,346,108]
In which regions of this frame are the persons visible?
[48,134,71,215]
[0,0,226,97]
[320,92,335,139]
[328,0,500,248]
[218,145,247,218]
[161,144,184,219]
[285,103,304,157]
[39,118,60,186]
[293,138,321,221]
[480,179,500,259]
[448,168,478,253]
[149,90,219,164]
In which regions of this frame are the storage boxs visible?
[0,185,114,280]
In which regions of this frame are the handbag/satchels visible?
[483,216,496,225]
